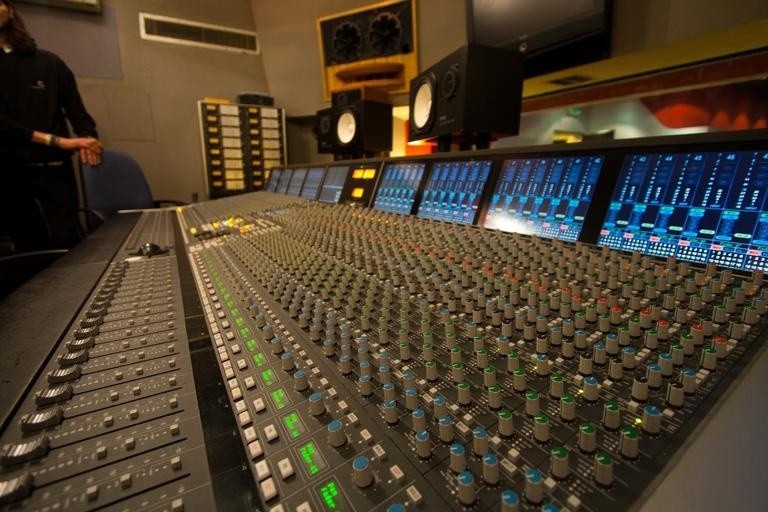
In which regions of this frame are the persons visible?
[0,0,103,251]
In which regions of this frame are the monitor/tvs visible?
[466,0,615,79]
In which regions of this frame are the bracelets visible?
[49,134,56,149]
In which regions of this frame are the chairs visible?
[0,135,92,303]
[78,149,188,237]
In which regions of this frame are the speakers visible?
[407,43,524,152]
[316,100,393,161]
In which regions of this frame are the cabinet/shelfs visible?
[197,99,288,198]
